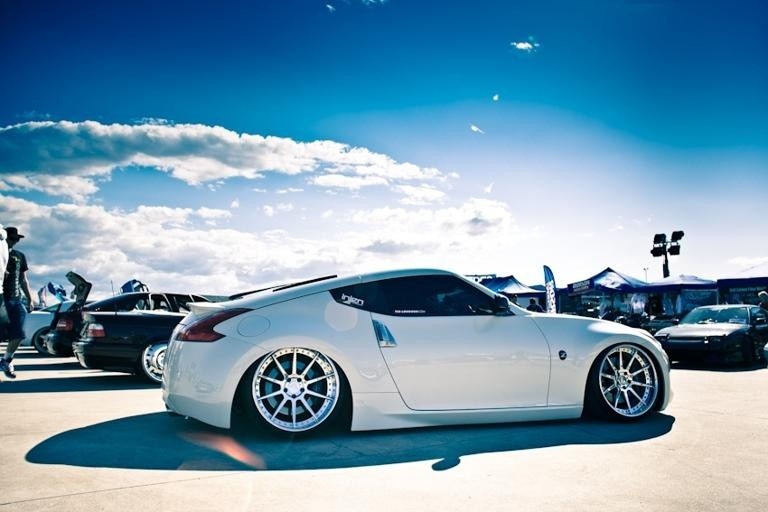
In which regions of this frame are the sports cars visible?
[159,266,672,439]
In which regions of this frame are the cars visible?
[41,269,218,359]
[653,304,768,369]
[15,298,107,358]
[73,305,219,387]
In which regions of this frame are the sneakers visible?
[0,357,16,378]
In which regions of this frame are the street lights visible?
[653,231,683,279]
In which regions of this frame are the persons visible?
[758,291,768,310]
[510,295,521,307]
[0,224,9,309]
[644,297,657,317]
[0,227,34,378]
[526,298,544,312]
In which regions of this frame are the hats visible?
[5,227,24,240]
